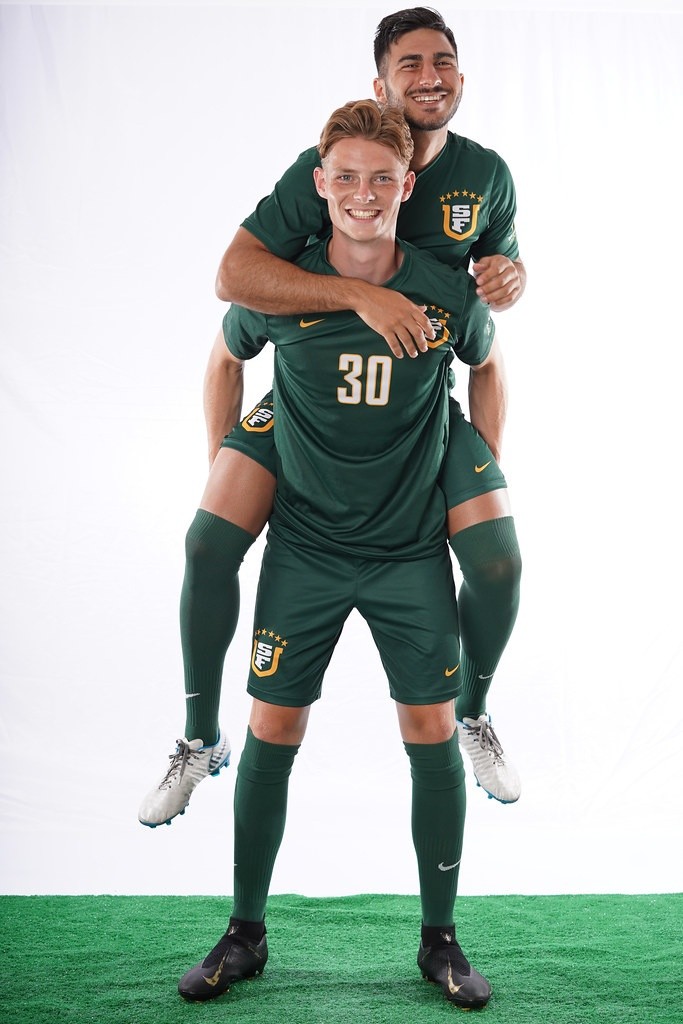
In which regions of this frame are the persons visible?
[141,5,528,827]
[175,98,504,1007]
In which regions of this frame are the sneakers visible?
[137,727,231,829]
[455,712,521,804]
[176,925,269,1003]
[417,938,494,1011]
